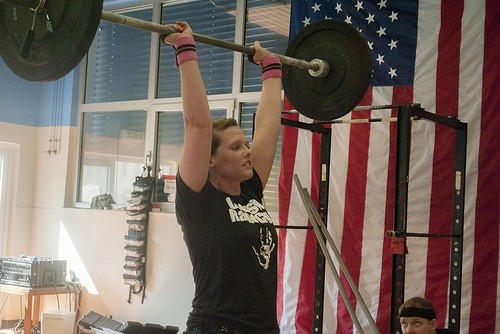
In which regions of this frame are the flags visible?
[276,0,500,334]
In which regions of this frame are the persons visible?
[161,21,282,334]
[399,297,459,334]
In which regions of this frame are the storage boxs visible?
[41,310,76,334]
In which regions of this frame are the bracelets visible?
[172,36,198,67]
[260,56,282,81]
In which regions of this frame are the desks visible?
[0,282,82,334]
